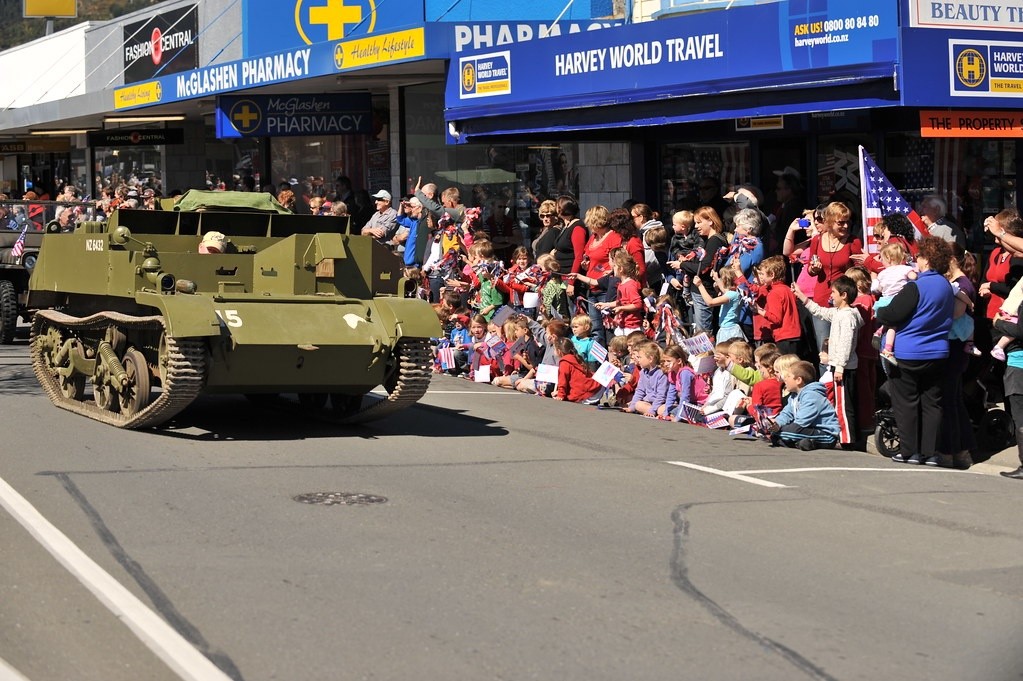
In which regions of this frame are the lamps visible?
[29,114,187,136]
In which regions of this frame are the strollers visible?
[871,351,1017,459]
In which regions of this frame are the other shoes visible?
[892,449,972,468]
[798,439,817,450]
[1000,466,1023,479]
[990,345,1005,361]
[964,344,982,356]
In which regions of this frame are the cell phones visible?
[798,220,809,227]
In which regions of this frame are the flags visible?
[10,225,27,258]
[591,361,619,387]
[535,361,559,384]
[862,146,932,264]
[590,340,608,364]
[681,332,714,357]
[436,346,456,370]
[680,399,702,423]
[705,414,729,430]
[487,333,507,355]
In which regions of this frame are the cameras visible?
[813,254,820,266]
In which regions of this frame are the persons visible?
[0,160,1023,479]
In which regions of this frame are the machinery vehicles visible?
[24,188,444,432]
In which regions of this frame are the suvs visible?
[0,198,97,343]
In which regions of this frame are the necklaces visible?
[827,230,842,287]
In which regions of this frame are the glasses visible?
[496,205,507,209]
[833,220,850,226]
[540,214,552,218]
[874,234,884,240]
[310,206,316,209]
[814,216,823,223]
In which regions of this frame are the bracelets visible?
[802,298,807,305]
[810,269,816,275]
[696,281,701,287]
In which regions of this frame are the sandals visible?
[871,330,881,350]
[879,349,897,366]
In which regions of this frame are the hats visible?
[140,189,154,197]
[399,194,414,202]
[126,191,138,196]
[202,231,227,253]
[28,199,46,218]
[372,189,391,202]
